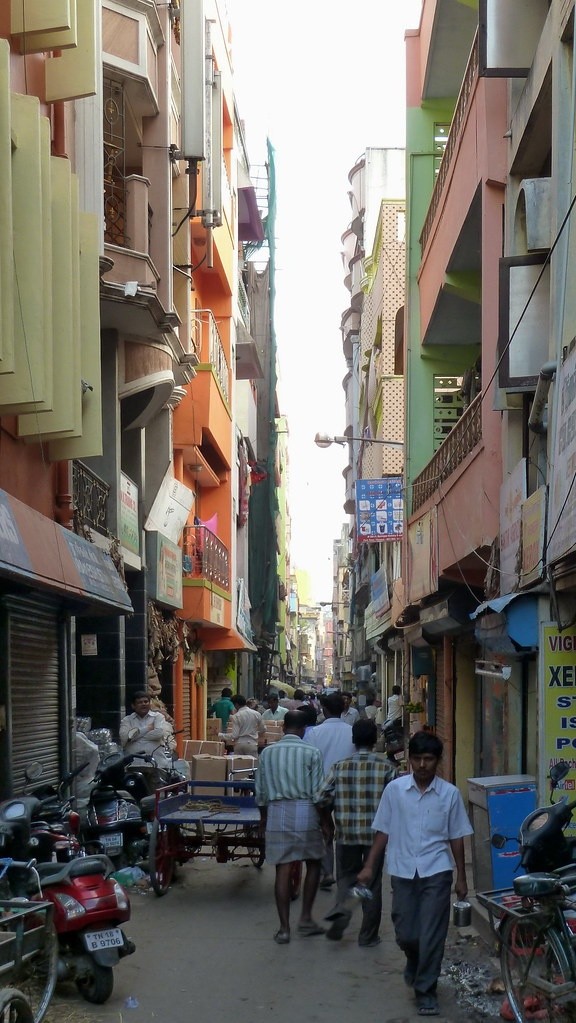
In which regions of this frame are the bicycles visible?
[474,871,576,1023]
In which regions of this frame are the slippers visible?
[320,877,337,888]
[273,930,290,944]
[297,922,326,937]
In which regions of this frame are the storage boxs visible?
[182,718,285,795]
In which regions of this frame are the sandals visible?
[404,958,418,988]
[417,991,440,1014]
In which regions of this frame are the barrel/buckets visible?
[453,901,472,927]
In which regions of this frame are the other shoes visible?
[325,917,350,940]
[363,936,381,947]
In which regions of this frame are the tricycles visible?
[147,767,303,902]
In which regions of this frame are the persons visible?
[210,682,403,761]
[120,691,176,769]
[254,710,333,944]
[313,718,401,946]
[304,694,354,888]
[358,731,475,1015]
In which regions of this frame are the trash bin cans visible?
[469,774,536,892]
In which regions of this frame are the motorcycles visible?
[489,761,576,1023]
[1,727,195,1023]
